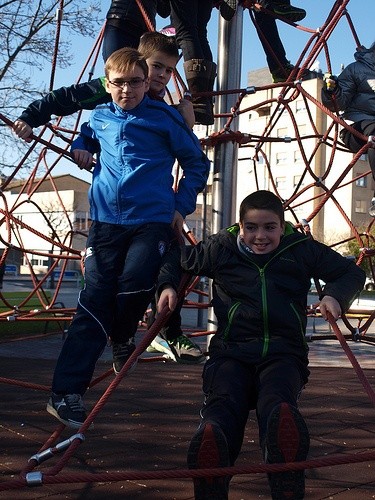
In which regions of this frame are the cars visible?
[309,276,375,293]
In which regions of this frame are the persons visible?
[157,190,366,500]
[44,49,211,430]
[13,0,375,358]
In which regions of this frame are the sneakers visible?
[271,65,319,83]
[45,392,96,432]
[260,0,307,23]
[185,420,236,499]
[150,333,204,363]
[263,402,311,500]
[110,334,138,375]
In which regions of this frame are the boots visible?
[167,59,217,125]
